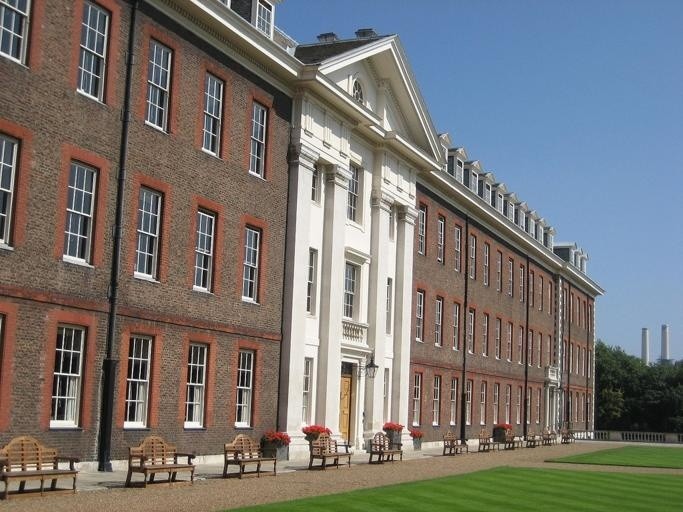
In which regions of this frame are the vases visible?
[413,438,423,450]
[385,429,402,450]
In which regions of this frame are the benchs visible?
[443,427,578,456]
[223,432,403,479]
[1,435,79,500]
[125,435,196,487]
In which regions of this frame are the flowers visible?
[382,422,405,431]
[495,423,513,430]
[302,424,333,436]
[409,429,424,440]
